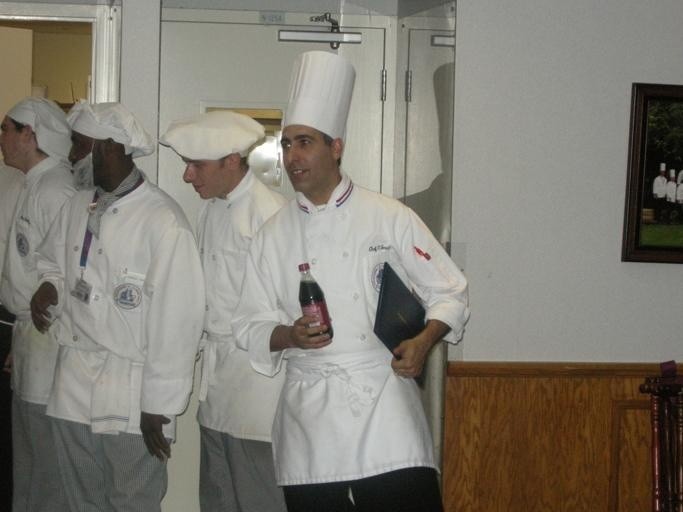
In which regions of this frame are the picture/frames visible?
[622,82,683,265]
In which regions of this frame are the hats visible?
[66,98,155,157]
[670,169,675,177]
[660,163,666,171]
[158,111,266,160]
[282,50,356,139]
[7,96,72,162]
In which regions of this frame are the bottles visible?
[297,262,334,343]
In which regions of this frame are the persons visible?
[25,96,204,511]
[0,96,77,512]
[675,179,683,224]
[157,106,289,511]
[651,161,667,224]
[231,124,469,511]
[664,168,676,223]
